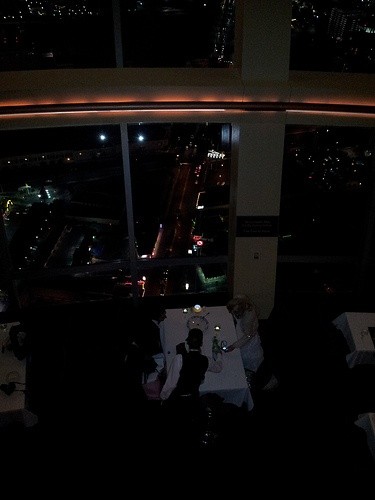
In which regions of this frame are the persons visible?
[226,294,265,372]
[159,329,209,401]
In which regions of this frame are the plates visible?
[6,370,20,383]
[186,316,209,332]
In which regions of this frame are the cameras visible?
[0,382,16,395]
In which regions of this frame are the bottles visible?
[211,336,222,363]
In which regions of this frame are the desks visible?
[0,322,37,428]
[331,311,375,369]
[155,309,255,412]
[356,413,375,459]
